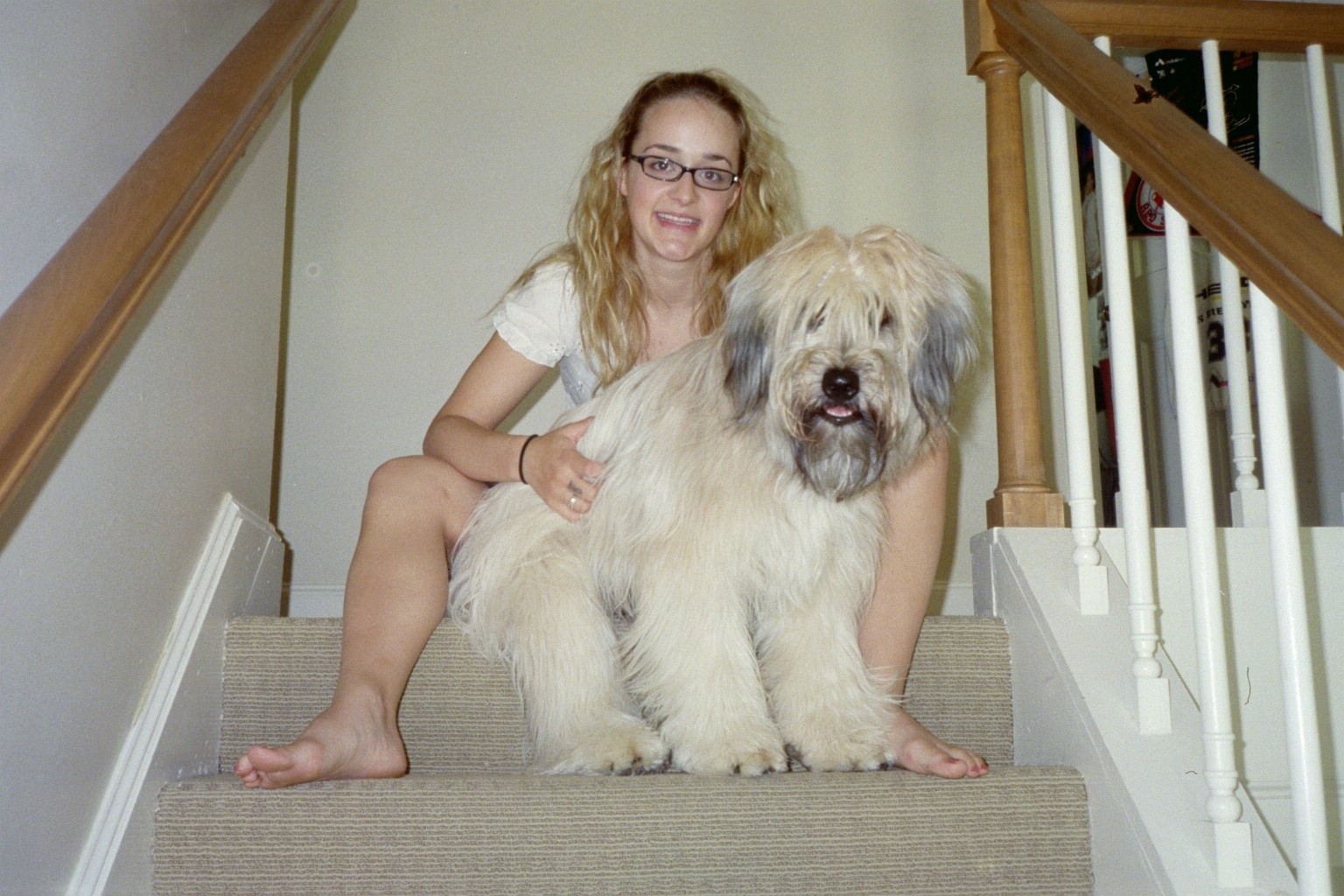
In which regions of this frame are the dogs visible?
[448,224,978,774]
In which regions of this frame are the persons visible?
[238,67,990,792]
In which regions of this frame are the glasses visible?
[620,150,742,192]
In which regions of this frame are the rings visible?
[568,492,580,510]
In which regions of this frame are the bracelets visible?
[518,434,539,484]
